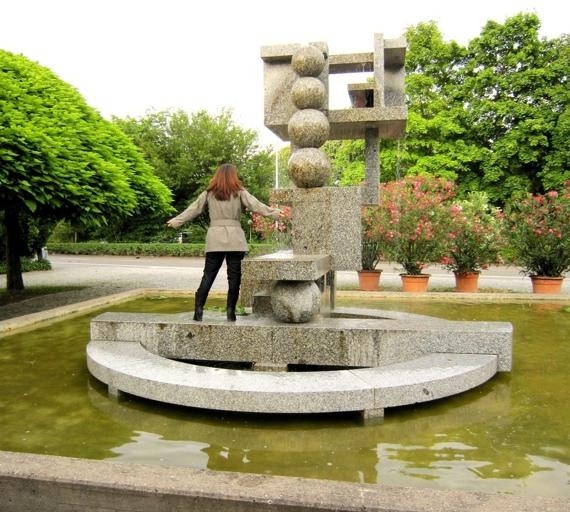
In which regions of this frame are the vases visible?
[355,271,564,299]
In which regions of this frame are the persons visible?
[167,163,286,322]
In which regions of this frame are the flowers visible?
[356,179,568,275]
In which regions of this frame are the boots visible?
[193,292,208,321]
[226,291,239,321]
